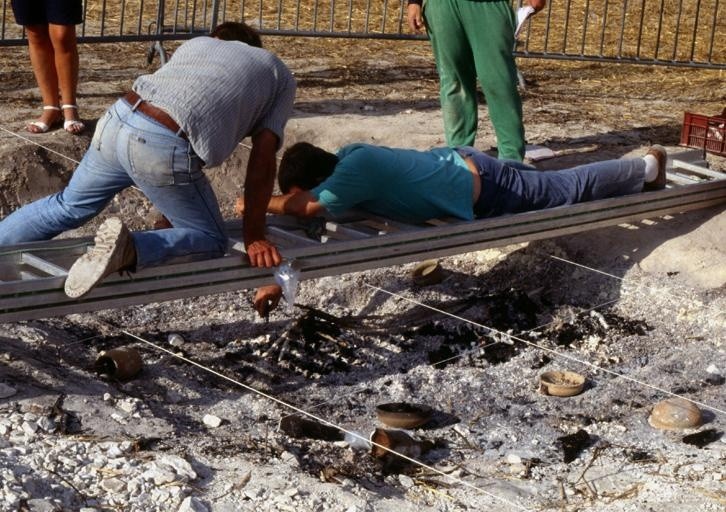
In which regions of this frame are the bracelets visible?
[408,0,423,7]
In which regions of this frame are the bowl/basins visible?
[411,260,443,285]
[540,370,585,397]
[648,399,703,430]
[376,402,432,429]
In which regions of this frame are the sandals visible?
[60,104,85,135]
[27,105,61,134]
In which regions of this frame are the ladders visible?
[0,147,726,323]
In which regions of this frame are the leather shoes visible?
[64,216,132,299]
[643,143,666,190]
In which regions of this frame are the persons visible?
[235,141,668,318]
[10,1,88,136]
[1,20,296,303]
[407,0,546,164]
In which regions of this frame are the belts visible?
[122,89,184,139]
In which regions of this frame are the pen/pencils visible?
[266,300,269,324]
